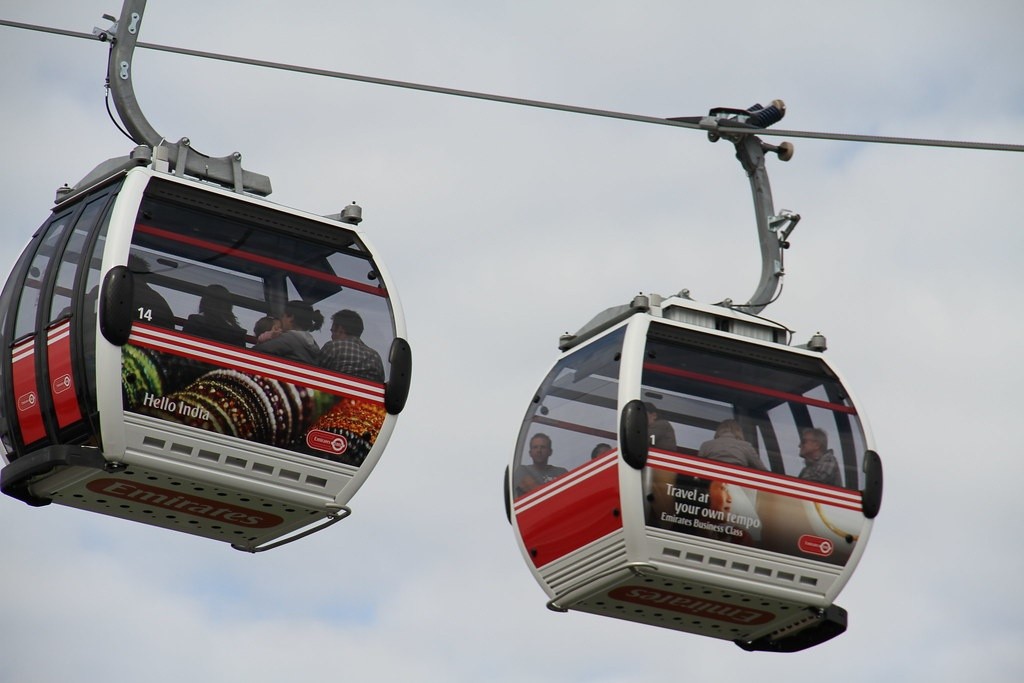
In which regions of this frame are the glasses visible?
[802,438,814,444]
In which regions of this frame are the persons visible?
[796,428,843,487]
[179,284,249,350]
[319,308,386,384]
[591,443,612,460]
[251,299,324,368]
[515,433,568,501]
[644,401,676,452]
[696,419,769,472]
[80,254,174,330]
[253,315,282,344]
[674,472,753,546]
